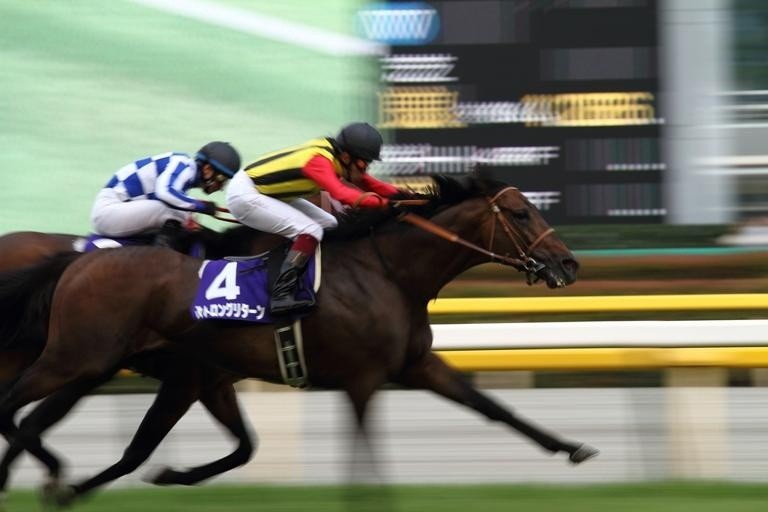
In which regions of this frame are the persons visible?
[227,121,411,316]
[91,141,241,257]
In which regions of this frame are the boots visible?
[269,247,314,312]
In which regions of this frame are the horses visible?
[0,171,600,510]
[1,225,476,508]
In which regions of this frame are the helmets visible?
[195,140,242,179]
[334,121,384,162]
